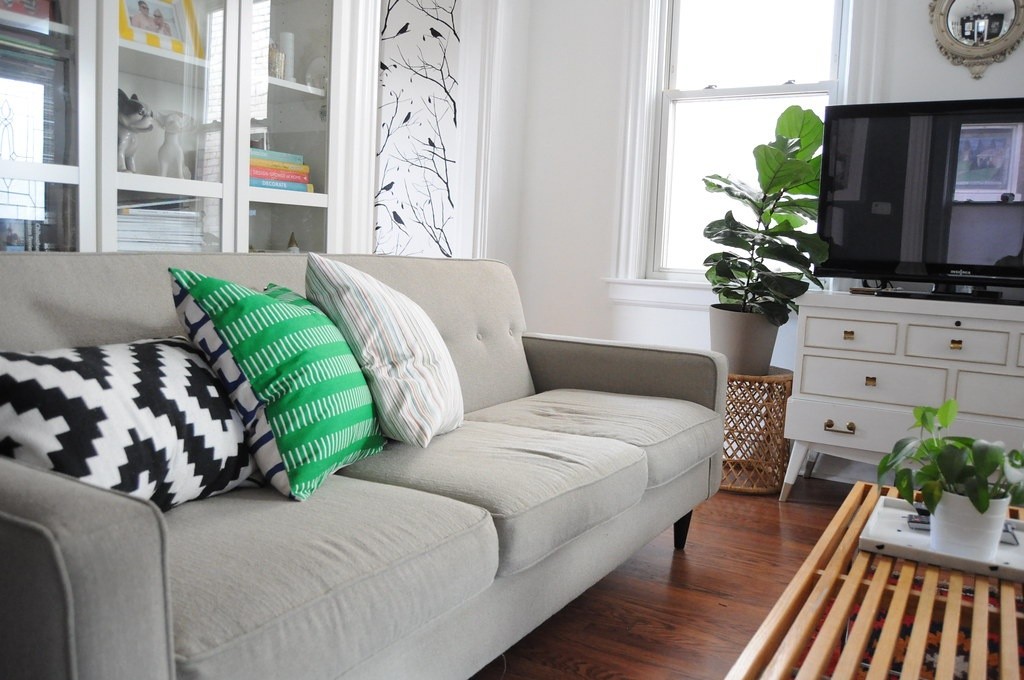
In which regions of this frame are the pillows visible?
[167,268,388,502]
[0,336,259,514]
[305,251,465,448]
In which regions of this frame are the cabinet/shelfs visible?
[778,288,1024,501]
[0,0,345,254]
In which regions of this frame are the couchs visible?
[0,250,729,680]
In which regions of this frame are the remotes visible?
[908,514,931,529]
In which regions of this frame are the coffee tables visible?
[723,480,1024,680]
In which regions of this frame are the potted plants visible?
[874,399,1024,561]
[700,106,829,376]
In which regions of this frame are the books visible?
[249,148,315,192]
[117,198,204,254]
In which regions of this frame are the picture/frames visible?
[117,0,202,58]
[953,123,1023,194]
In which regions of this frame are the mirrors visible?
[928,0,1024,81]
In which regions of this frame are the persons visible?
[130,1,156,31]
[153,10,171,35]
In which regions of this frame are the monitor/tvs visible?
[814,97,1024,306]
